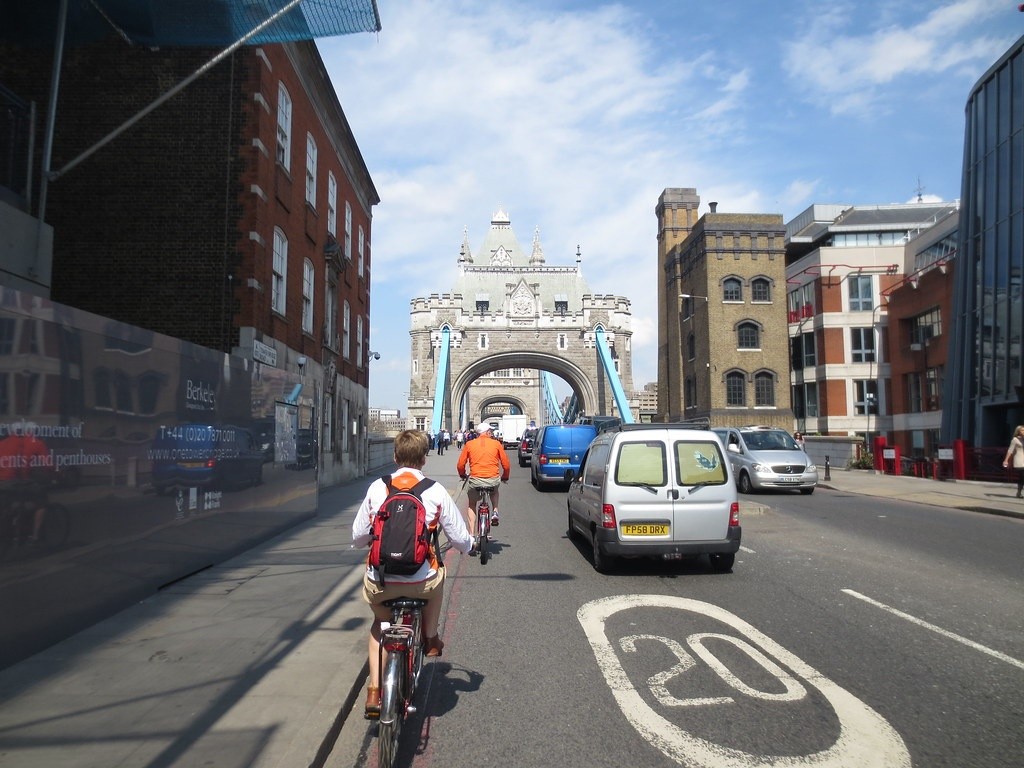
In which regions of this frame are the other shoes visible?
[468,549,477,556]
[491,511,499,526]
[1017,492,1023,498]
[424,633,444,657]
[365,686,381,712]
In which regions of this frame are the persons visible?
[457,423,510,556]
[791,432,806,454]
[353,428,475,718]
[0,423,57,550]
[425,428,504,456]
[1002,425,1024,498]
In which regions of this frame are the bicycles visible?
[476,488,491,563]
[378,597,419,768]
[1,500,73,563]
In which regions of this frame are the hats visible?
[477,422,496,434]
[11,420,37,436]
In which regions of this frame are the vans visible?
[709,427,818,496]
[563,430,741,570]
[530,422,605,491]
[151,420,267,498]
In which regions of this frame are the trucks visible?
[501,414,527,452]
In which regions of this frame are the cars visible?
[517,429,552,466]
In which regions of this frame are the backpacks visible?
[368,474,437,587]
